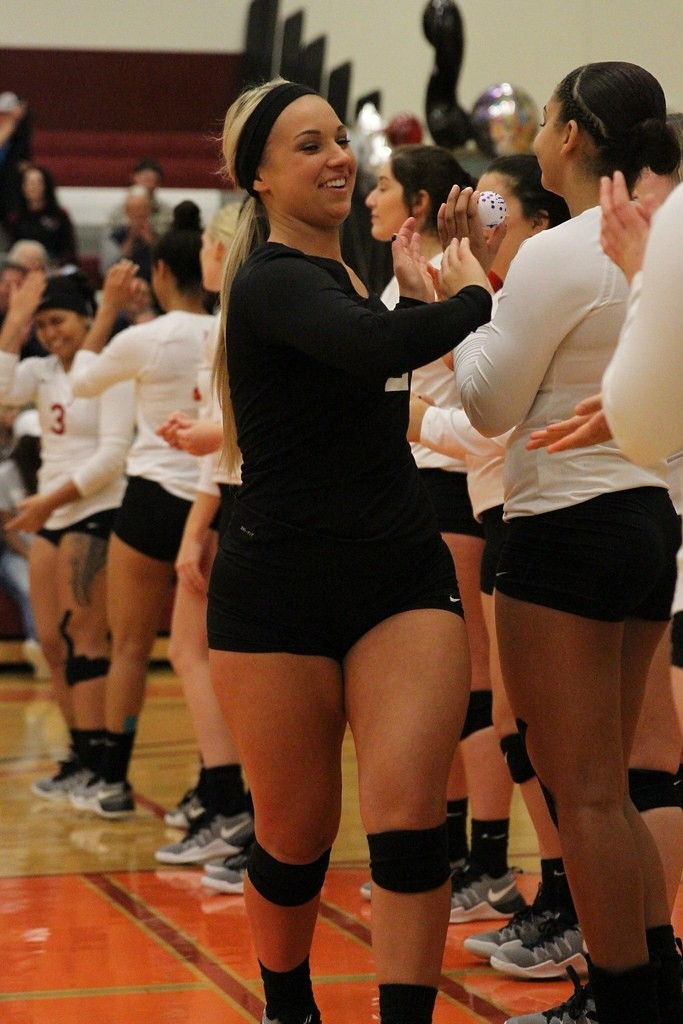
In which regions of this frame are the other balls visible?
[475,188,508,229]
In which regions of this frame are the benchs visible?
[30,128,234,192]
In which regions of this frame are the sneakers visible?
[201,810,254,895]
[29,759,95,800]
[503,965,683,1024]
[261,1006,323,1024]
[450,867,529,924]
[461,882,592,981]
[163,789,207,832]
[360,882,371,902]
[67,775,137,820]
[151,809,255,865]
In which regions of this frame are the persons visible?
[0,63,683,1024]
[207,78,492,1024]
